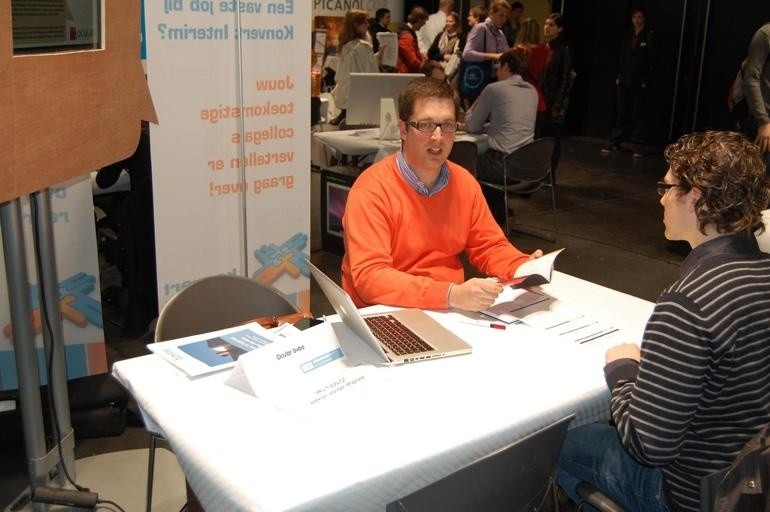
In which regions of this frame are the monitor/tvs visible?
[346,73,426,140]
[11,0,102,55]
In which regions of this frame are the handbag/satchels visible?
[728,56,770,133]
[460,61,491,98]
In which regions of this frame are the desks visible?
[110,262,657,512]
[312,122,492,174]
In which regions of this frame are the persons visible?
[600,9,657,158]
[726,23,770,155]
[559,130,770,512]
[340,78,545,313]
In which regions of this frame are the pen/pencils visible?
[464,320,505,329]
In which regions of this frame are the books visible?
[495,246,566,291]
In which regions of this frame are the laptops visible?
[304,258,473,365]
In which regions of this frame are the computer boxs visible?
[321,165,366,256]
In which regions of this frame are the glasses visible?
[657,182,680,195]
[406,121,460,132]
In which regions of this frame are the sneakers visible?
[632,146,645,158]
[601,145,620,153]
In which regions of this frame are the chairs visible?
[445,139,482,183]
[142,271,306,512]
[574,425,770,512]
[477,137,560,249]
